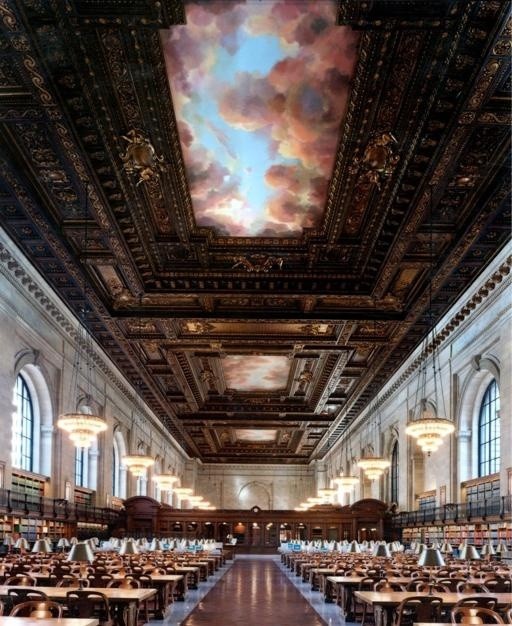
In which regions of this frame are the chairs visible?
[0,549,233,626]
[277,549,512,626]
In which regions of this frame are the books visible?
[386,478,511,558]
[1,470,120,545]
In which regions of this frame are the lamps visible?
[288,539,507,596]
[57,176,112,453]
[147,347,217,511]
[3,536,219,590]
[118,294,156,480]
[295,311,455,512]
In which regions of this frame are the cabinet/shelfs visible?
[401,521,512,548]
[0,512,121,555]
